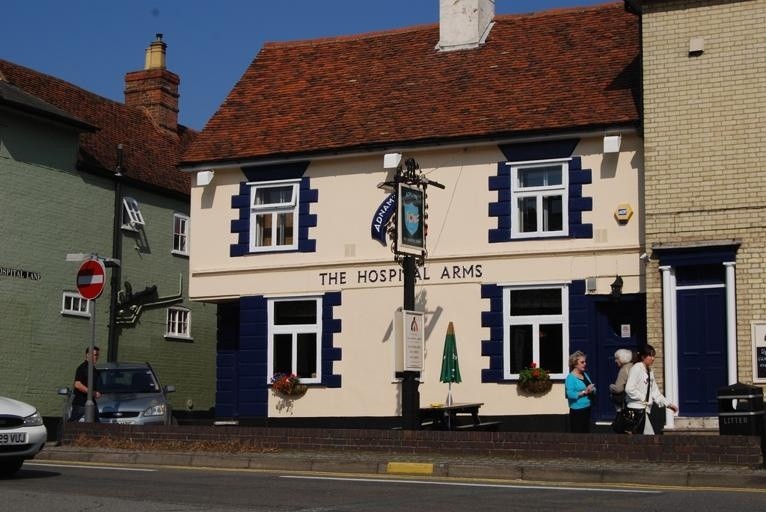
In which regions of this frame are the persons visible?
[65,344,103,422]
[608,349,635,409]
[564,350,600,432]
[621,342,680,434]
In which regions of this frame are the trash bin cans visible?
[717,383,766,436]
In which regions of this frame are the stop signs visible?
[75,259,106,300]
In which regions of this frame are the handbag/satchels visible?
[612,408,645,434]
[608,384,626,402]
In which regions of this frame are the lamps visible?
[604,133,621,153]
[197,170,214,186]
[384,153,402,168]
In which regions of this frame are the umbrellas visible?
[439,320,464,427]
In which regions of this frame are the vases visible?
[277,386,306,396]
[526,379,553,394]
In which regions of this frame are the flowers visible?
[518,363,548,387]
[270,371,299,392]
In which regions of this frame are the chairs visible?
[132,372,150,386]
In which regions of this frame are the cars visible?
[57,362,176,438]
[0,396,48,474]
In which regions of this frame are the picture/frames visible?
[751,319,766,384]
